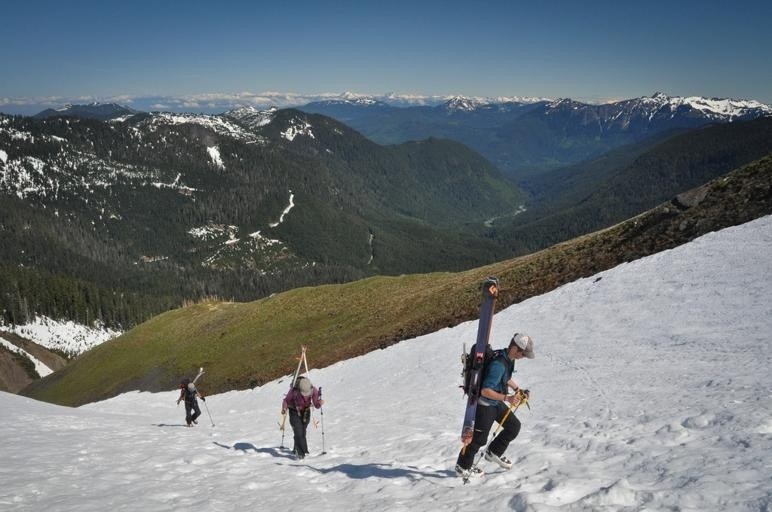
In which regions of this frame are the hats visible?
[187,383,196,392]
[299,378,311,397]
[513,333,535,359]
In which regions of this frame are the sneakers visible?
[456,463,475,477]
[485,449,512,469]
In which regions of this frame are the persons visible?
[282,377,324,457]
[455,334,536,476]
[176,382,206,427]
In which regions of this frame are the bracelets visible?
[514,386,519,392]
[502,394,508,403]
